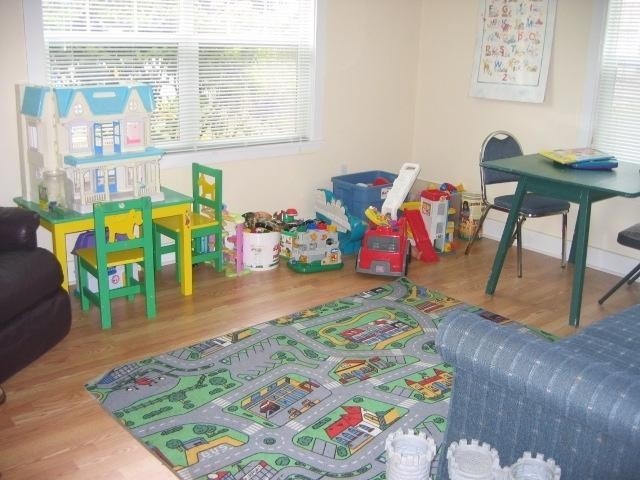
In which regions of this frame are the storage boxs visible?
[331,170,399,222]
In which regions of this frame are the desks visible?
[12,187,194,299]
[478,150,640,327]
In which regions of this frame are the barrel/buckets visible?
[242,230,281,272]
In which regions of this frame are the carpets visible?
[83,275,559,480]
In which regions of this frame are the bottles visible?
[38,170,75,219]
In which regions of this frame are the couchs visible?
[435,305,639,480]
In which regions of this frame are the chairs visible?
[74,195,157,332]
[598,223,639,305]
[465,129,571,279]
[152,162,226,282]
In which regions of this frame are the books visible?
[536,143,614,166]
[551,159,618,170]
